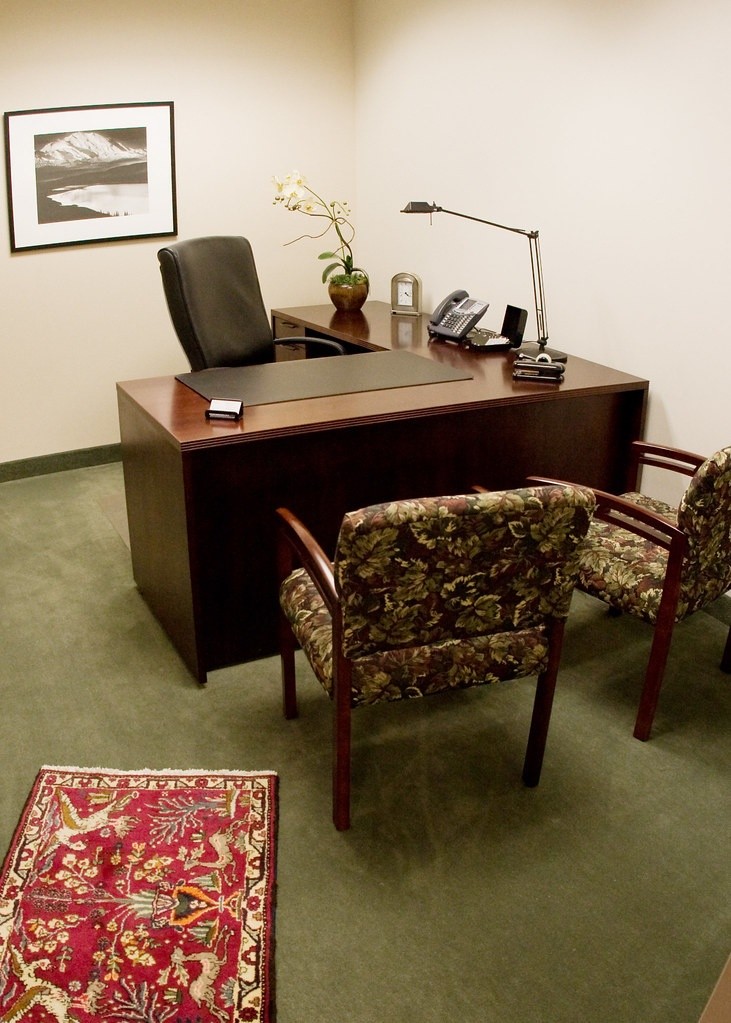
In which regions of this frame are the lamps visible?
[399,203,569,365]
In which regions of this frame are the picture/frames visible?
[2,101,178,252]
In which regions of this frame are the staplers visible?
[512,361,565,383]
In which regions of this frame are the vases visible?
[328,283,369,314]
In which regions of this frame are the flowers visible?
[271,169,370,291]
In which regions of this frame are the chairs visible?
[525,440,731,742]
[271,484,598,829]
[158,236,346,374]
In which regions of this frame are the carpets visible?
[0,764,280,1023]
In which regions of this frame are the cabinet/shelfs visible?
[116,301,649,687]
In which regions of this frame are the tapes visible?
[535,353,551,364]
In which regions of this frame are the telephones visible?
[426,288,490,343]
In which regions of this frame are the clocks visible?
[390,273,423,319]
